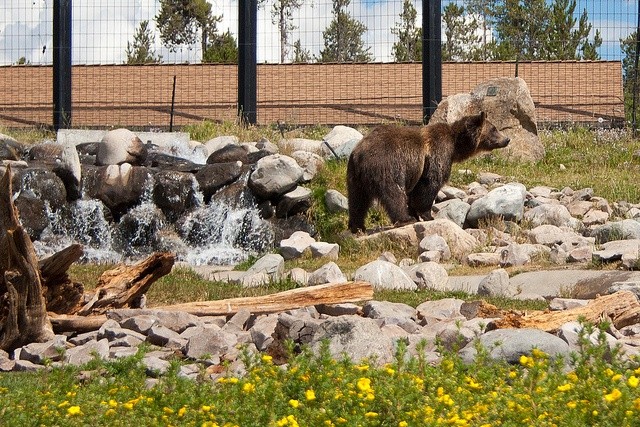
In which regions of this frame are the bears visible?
[346,111,510,233]
